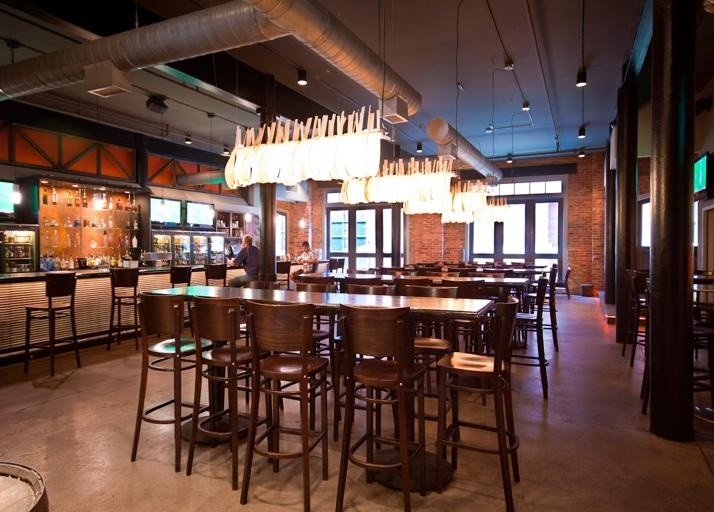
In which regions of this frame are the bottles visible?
[217,213,239,228]
[38,185,141,270]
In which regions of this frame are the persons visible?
[226,235,261,288]
[289,240,319,284]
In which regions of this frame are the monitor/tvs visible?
[185,199,215,230]
[230,244,241,256]
[692,150,713,202]
[0,180,20,221]
[150,195,183,228]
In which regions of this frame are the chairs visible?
[630,268,713,436]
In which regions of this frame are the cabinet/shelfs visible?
[0,173,143,275]
